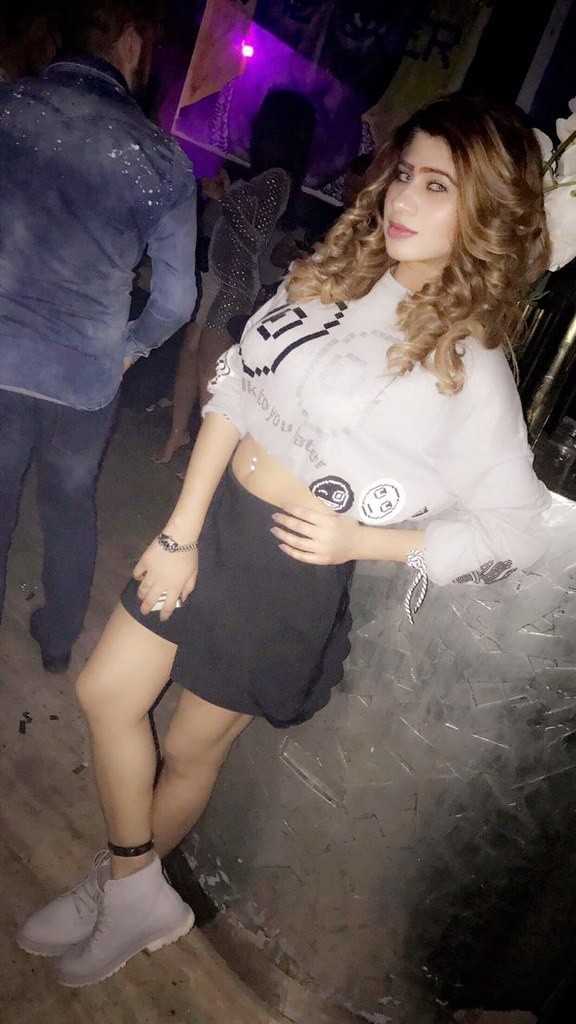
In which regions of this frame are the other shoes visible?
[30,608,70,672]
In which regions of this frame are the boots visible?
[57,851,196,986]
[16,850,111,957]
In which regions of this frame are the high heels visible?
[152,431,190,464]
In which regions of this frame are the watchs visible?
[159,532,199,552]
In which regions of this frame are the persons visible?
[151,92,317,483]
[16,90,549,988]
[0,0,197,673]
